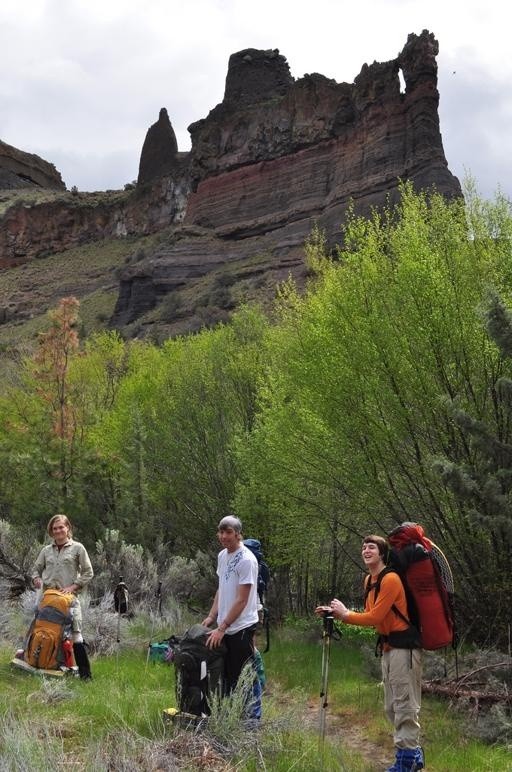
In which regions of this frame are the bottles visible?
[63,637,74,667]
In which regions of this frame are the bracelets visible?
[223,620,231,627]
[217,625,226,633]
[208,616,215,621]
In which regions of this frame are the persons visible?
[30,514,94,684]
[199,514,262,729]
[313,533,426,771]
[242,539,270,687]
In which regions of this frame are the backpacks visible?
[361,520,460,652]
[242,537,271,605]
[172,622,230,718]
[23,586,78,671]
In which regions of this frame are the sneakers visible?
[384,745,426,772]
